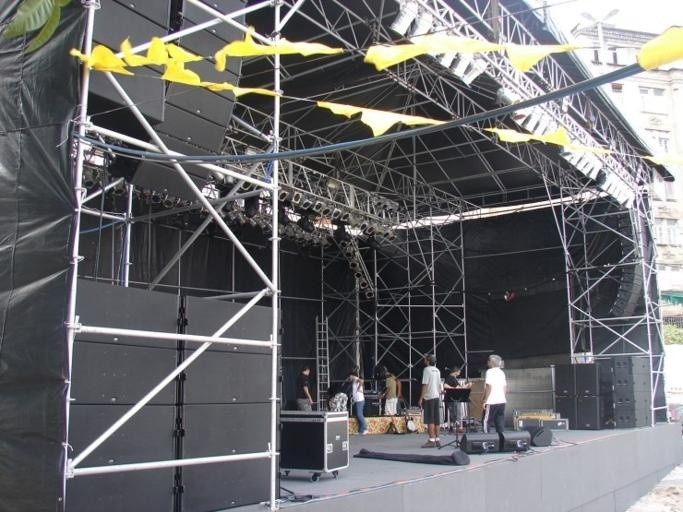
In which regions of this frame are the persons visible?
[294,364,314,411]
[442,365,472,433]
[374,365,402,416]
[347,372,368,434]
[479,354,507,434]
[416,353,441,448]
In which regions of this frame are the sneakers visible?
[421,441,440,448]
[353,430,368,435]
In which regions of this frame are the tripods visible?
[438,403,461,449]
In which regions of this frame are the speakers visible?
[554,363,614,430]
[529,427,552,446]
[460,432,499,453]
[609,210,644,321]
[500,430,532,450]
[108,1,246,203]
